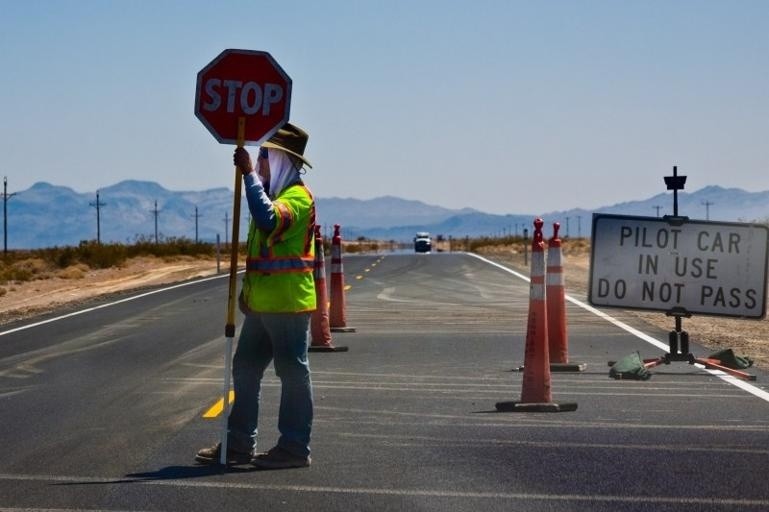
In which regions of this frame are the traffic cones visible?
[326,222,358,333]
[494,217,579,414]
[302,223,352,355]
[514,220,588,375]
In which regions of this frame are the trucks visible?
[412,230,434,253]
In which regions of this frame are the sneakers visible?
[250,445,311,468]
[195,443,251,464]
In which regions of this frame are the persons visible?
[192,117,320,473]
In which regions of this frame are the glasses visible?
[260,148,268,159]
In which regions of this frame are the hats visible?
[261,124,312,168]
